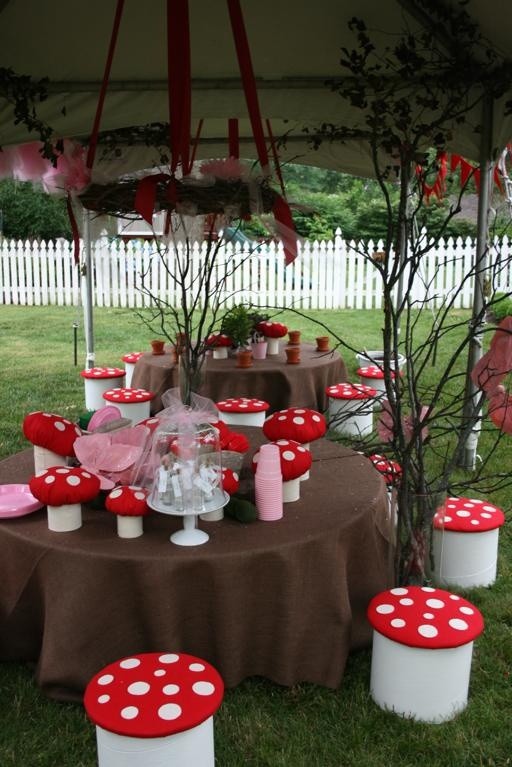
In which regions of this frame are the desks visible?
[130,336,347,419]
[1,423,398,706]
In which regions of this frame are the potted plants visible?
[221,304,260,368]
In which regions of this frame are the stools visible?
[122,353,146,387]
[101,388,150,430]
[84,651,223,767]
[216,397,269,432]
[357,366,396,410]
[80,366,126,413]
[366,453,505,725]
[324,381,376,435]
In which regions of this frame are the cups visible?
[254,444,285,523]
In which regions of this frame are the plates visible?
[0,483,46,520]
[87,405,122,433]
